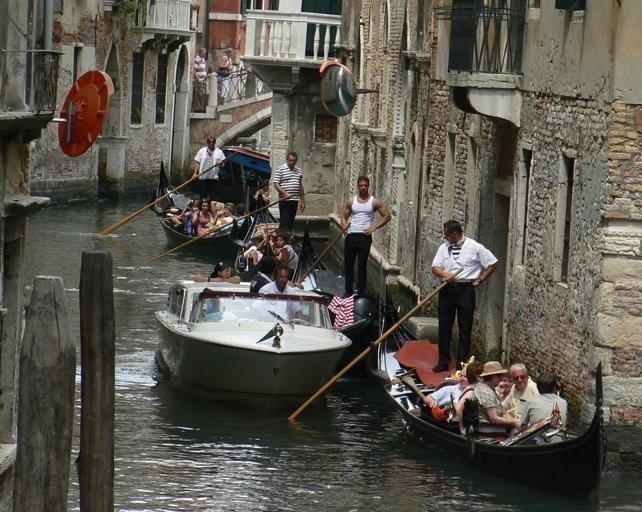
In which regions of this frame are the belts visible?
[448,283,469,286]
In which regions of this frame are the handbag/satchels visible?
[478,421,511,437]
[463,399,479,426]
[550,403,561,429]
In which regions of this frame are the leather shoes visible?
[342,290,354,299]
[432,365,448,372]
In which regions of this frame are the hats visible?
[224,202,235,214]
[479,361,508,376]
[250,231,264,241]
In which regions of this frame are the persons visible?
[170,179,269,237]
[340,175,392,299]
[192,227,304,322]
[423,359,569,447]
[272,151,306,240]
[191,135,226,209]
[193,47,210,113]
[218,47,233,103]
[432,219,498,372]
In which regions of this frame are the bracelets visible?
[477,278,484,284]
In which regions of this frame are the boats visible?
[151,160,253,256]
[152,279,352,396]
[223,144,272,176]
[379,285,606,489]
[235,178,378,371]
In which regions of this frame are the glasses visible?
[208,141,215,143]
[513,375,527,380]
